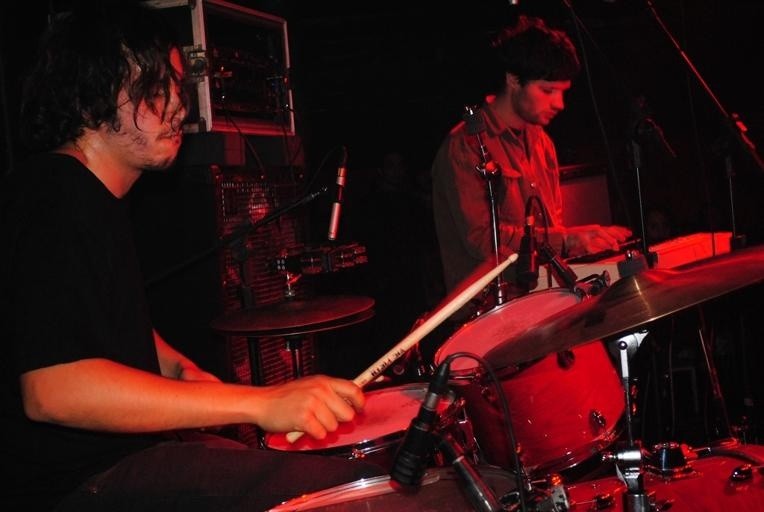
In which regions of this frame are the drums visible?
[269,470,481,511]
[434,290,630,482]
[258,383,466,478]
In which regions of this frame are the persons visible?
[430,16,634,330]
[2,0,364,512]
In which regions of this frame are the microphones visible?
[515,206,538,291]
[326,142,349,242]
[388,352,453,498]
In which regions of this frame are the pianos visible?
[537,230,732,290]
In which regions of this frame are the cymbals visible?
[480,250,764,372]
[211,296,379,338]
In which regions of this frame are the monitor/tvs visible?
[195,0,294,135]
